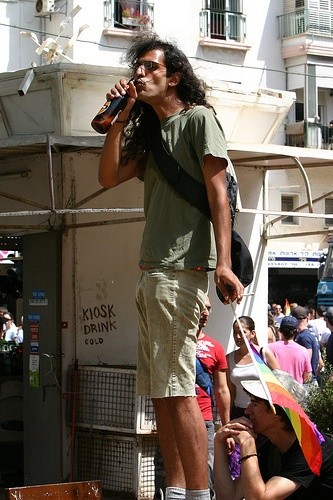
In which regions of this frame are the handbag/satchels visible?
[215,229,254,304]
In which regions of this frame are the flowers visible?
[122,7,150,25]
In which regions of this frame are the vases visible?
[122,17,145,26]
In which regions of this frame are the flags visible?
[285,299,291,317]
[248,342,322,476]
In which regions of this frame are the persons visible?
[97,32,243,500]
[194,297,333,500]
[0,307,23,350]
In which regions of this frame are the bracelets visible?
[239,453,257,464]
[116,120,126,123]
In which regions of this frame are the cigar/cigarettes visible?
[240,293,255,296]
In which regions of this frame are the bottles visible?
[91,78,136,134]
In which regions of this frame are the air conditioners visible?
[34,0,55,16]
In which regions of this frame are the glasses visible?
[133,61,166,71]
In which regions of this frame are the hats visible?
[291,306,308,318]
[240,369,307,408]
[323,307,333,317]
[282,315,298,328]
